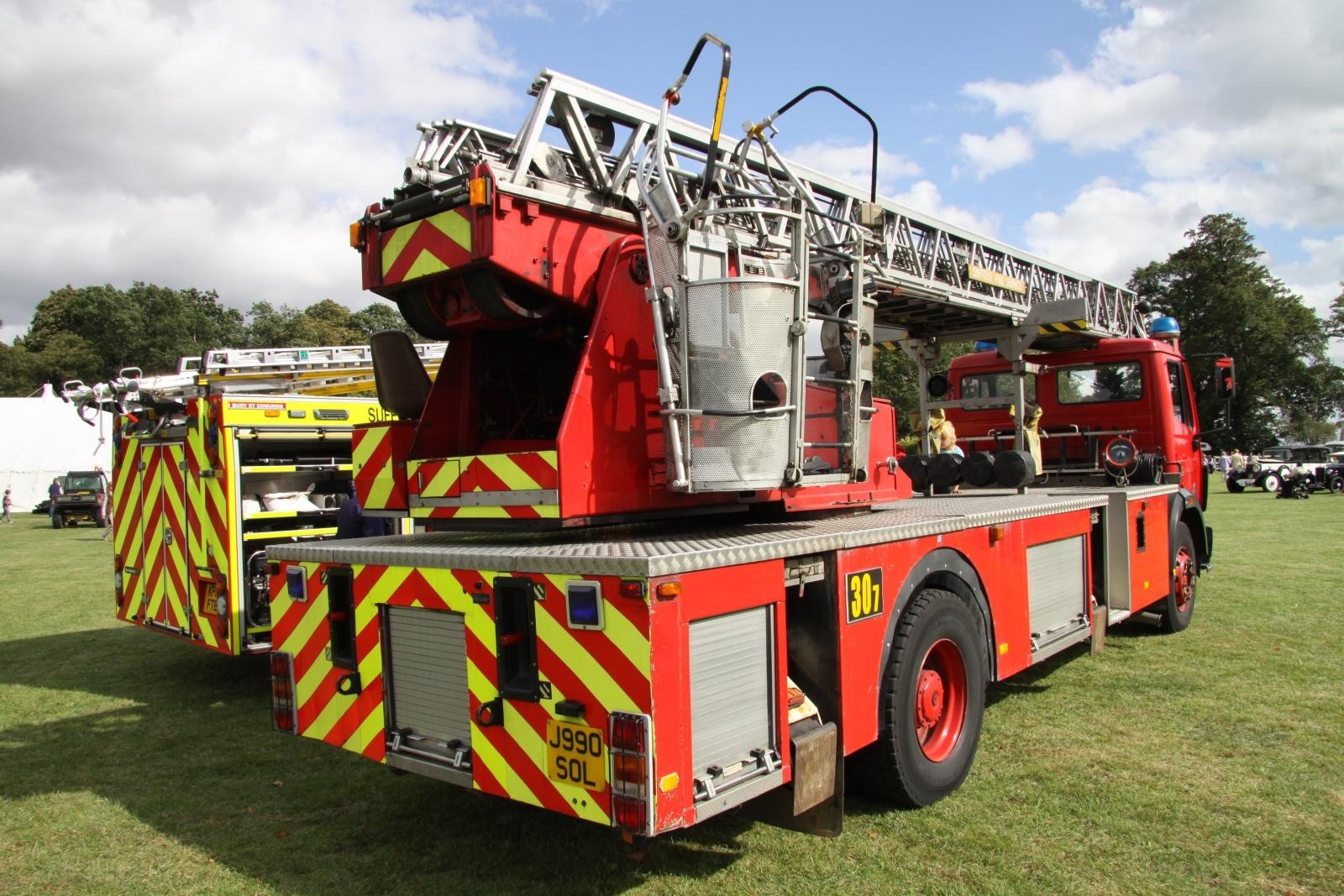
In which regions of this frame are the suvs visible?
[52,467,113,530]
[1225,445,1336,493]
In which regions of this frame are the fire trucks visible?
[55,29,1239,840]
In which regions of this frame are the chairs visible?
[1292,474,1308,492]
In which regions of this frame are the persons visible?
[48,477,63,520]
[1218,448,1263,483]
[938,421,965,495]
[0,489,15,523]
